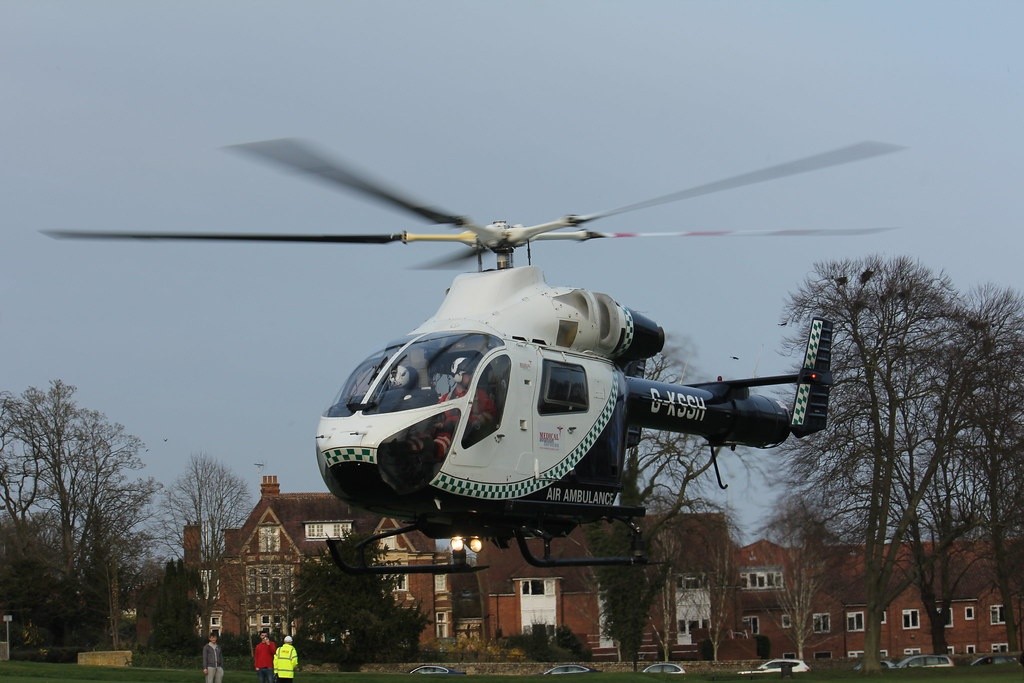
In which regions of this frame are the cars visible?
[408,665,466,675]
[538,664,603,675]
[853,660,897,670]
[737,658,811,674]
[640,663,688,675]
[896,655,956,668]
[970,655,1022,666]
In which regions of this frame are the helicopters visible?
[36,134,912,577]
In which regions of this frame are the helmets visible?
[388,365,410,387]
[448,357,470,383]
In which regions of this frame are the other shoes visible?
[398,465,427,481]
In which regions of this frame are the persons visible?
[254,631,277,683]
[273,636,298,683]
[388,358,497,464]
[203,633,225,683]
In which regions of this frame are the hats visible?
[283,636,293,643]
